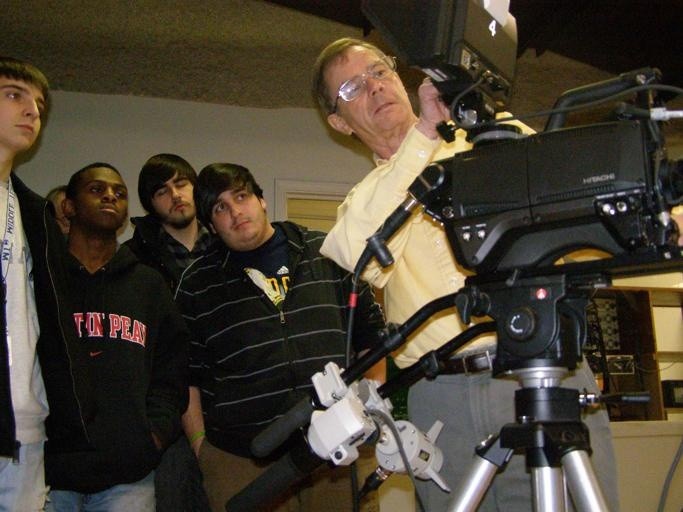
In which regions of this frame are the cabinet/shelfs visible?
[585,286,683,422]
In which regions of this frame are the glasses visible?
[332,55,397,113]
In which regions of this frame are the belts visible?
[441,352,496,375]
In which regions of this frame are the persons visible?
[172,163,387,511]
[313,37,619,511]
[48,185,69,228]
[122,154,209,509]
[0,61,65,511]
[46,163,176,511]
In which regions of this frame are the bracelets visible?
[189,432,204,443]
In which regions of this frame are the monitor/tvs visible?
[357,0,519,123]
